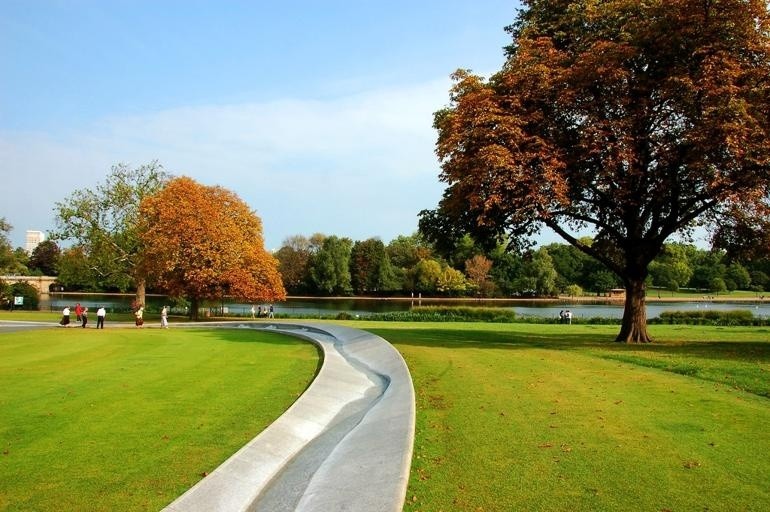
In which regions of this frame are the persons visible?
[257,305,262,318]
[269,304,274,319]
[134,305,144,328]
[62,306,70,328]
[264,306,268,318]
[82,307,88,328]
[560,310,572,324]
[411,292,413,298]
[251,305,255,318]
[419,293,421,298]
[74,303,82,321]
[160,305,169,329]
[96,307,106,329]
